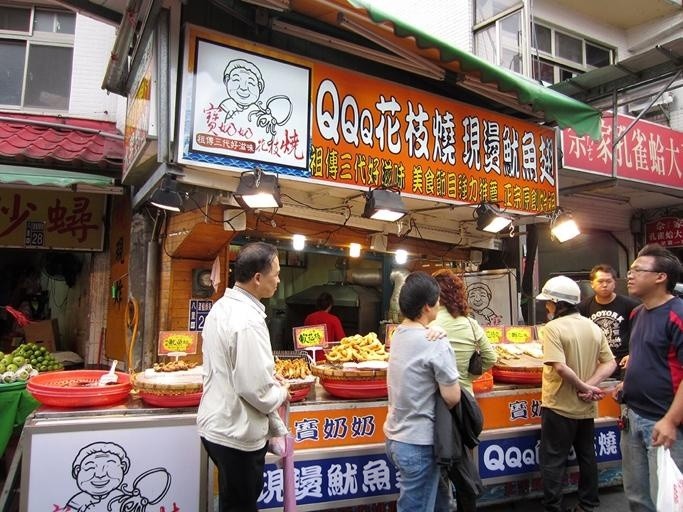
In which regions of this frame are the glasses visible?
[626,267,657,276]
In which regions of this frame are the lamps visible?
[147,173,184,211]
[547,205,582,244]
[233,168,282,212]
[472,202,513,235]
[360,185,409,223]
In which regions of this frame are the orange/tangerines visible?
[12,343,62,372]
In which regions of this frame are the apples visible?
[0,352,37,384]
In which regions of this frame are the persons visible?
[301,292,346,361]
[194,241,292,512]
[382,272,464,511]
[431,266,500,511]
[577,264,638,380]
[533,275,618,511]
[611,243,683,512]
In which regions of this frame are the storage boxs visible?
[23,319,61,352]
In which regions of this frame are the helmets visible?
[536,276,581,305]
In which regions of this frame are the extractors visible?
[284,282,383,308]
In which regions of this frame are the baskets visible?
[131,360,544,395]
